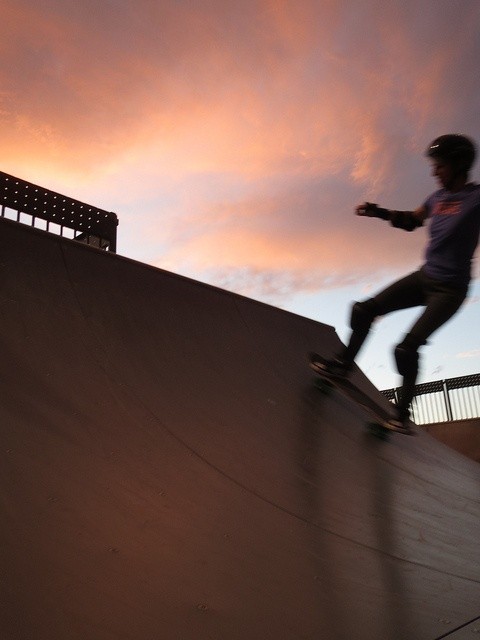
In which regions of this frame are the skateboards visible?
[306,350,418,438]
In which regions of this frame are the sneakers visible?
[310,353,352,380]
[388,411,410,428]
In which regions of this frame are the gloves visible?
[364,201,388,220]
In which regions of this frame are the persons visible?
[309,135,479,427]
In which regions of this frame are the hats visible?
[424,133,476,165]
